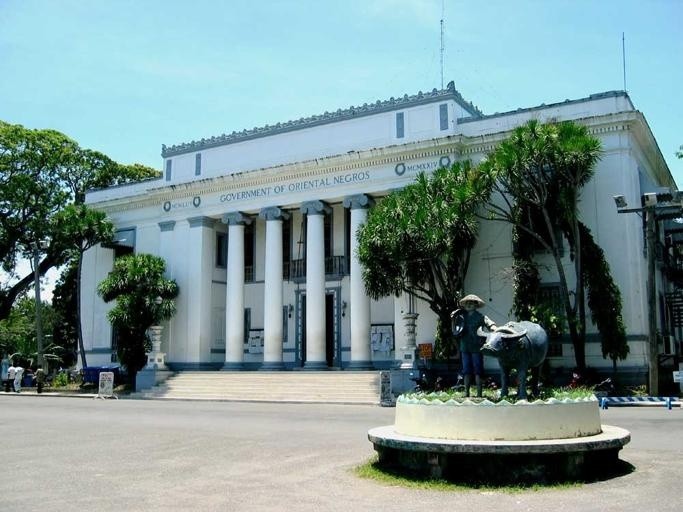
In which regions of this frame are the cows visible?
[477,321,548,400]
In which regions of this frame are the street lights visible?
[612,193,658,396]
[30,240,47,366]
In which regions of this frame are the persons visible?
[450,295,499,397]
[5,364,15,392]
[34,364,44,393]
[14,362,24,393]
